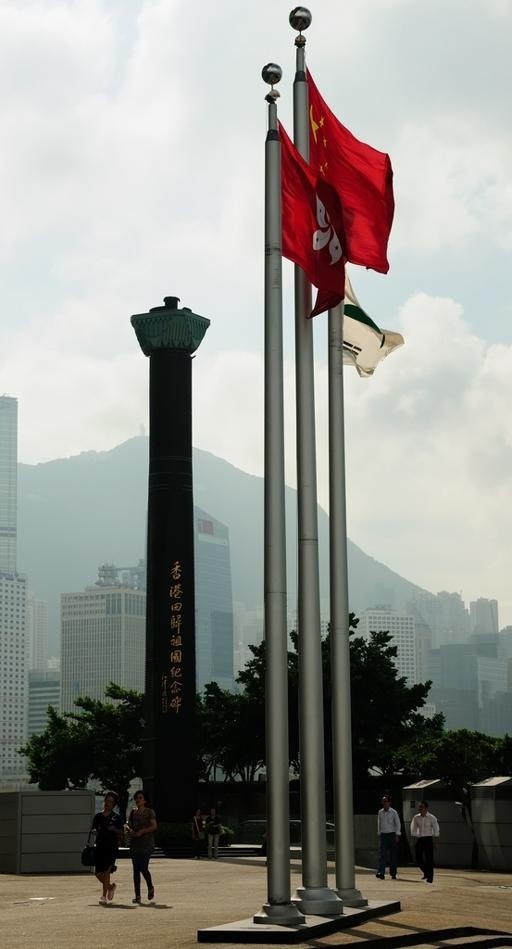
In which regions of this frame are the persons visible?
[191,807,205,861]
[204,807,222,861]
[375,794,402,881]
[125,790,158,904]
[79,792,125,906]
[410,800,441,884]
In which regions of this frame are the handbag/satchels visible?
[81,848,98,866]
[199,832,205,839]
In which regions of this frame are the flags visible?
[342,271,406,379]
[307,65,396,276]
[277,118,346,321]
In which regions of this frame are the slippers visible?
[132,898,142,903]
[98,883,116,904]
[148,886,154,900]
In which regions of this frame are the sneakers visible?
[376,873,384,880]
[392,875,396,879]
